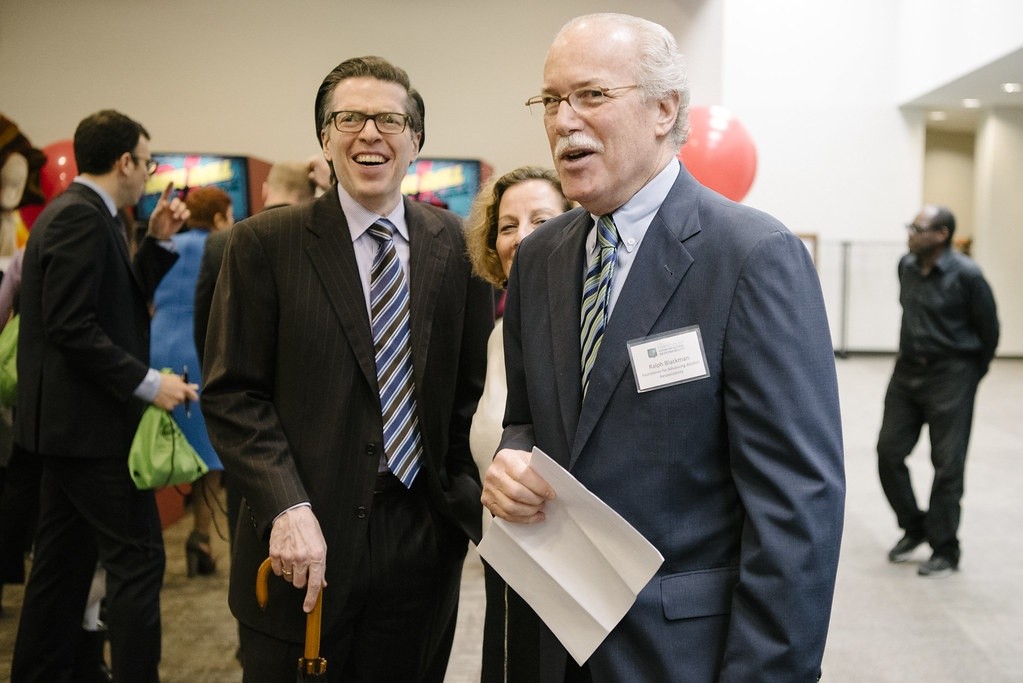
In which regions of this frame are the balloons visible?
[681,107,755,199]
[39,142,79,203]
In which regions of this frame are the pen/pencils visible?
[182,364,189,416]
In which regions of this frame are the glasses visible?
[325,110,414,134]
[132,157,159,175]
[524,84,638,118]
[906,225,938,234]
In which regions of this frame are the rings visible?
[282,568,292,574]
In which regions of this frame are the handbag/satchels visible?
[0,315,18,405]
[127,367,210,489]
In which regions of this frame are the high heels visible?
[187,532,216,577]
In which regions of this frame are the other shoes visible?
[889,518,930,562]
[918,548,959,578]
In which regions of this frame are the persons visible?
[194,156,328,360]
[1,115,46,616]
[465,166,579,478]
[10,109,199,683]
[201,55,494,683]
[148,186,235,574]
[480,12,844,683]
[875,206,1000,575]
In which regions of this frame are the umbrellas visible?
[258,556,328,683]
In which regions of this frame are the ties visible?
[367,219,425,490]
[580,215,619,404]
